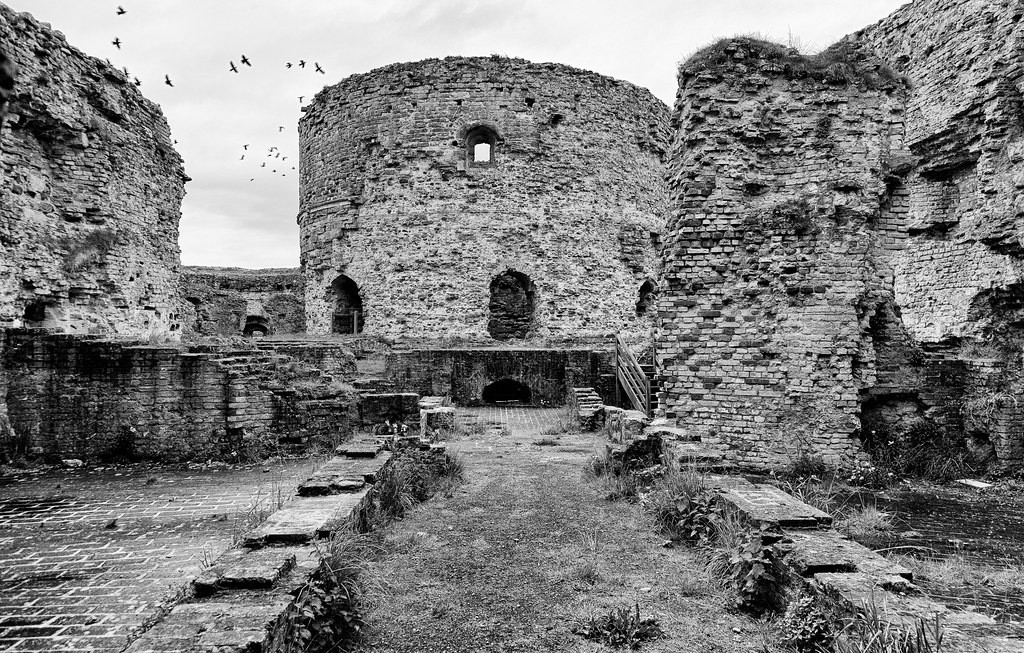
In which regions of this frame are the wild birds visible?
[228,53,252,75]
[163,73,176,91]
[114,4,128,17]
[104,56,144,89]
[173,138,179,148]
[111,35,124,50]
[285,59,327,75]
[297,95,305,106]
[239,124,296,182]
